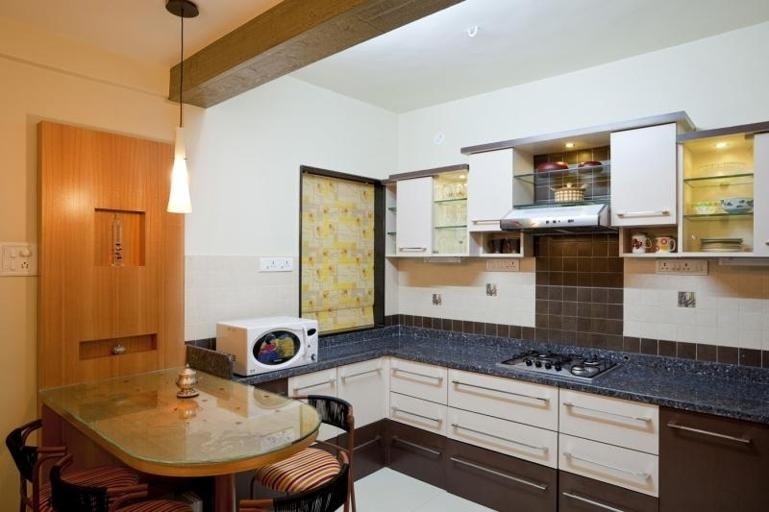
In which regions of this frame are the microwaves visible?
[215,316,318,377]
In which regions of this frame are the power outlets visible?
[2,244,38,277]
[485,259,502,272]
[258,258,277,272]
[681,261,709,274]
[503,259,519,272]
[656,260,681,274]
[277,258,293,272]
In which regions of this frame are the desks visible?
[39,365,321,512]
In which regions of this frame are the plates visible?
[701,238,745,253]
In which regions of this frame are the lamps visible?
[164,0,198,214]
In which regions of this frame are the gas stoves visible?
[494,349,623,385]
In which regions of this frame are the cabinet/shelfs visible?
[560,388,660,512]
[659,418,769,511]
[461,140,534,232]
[288,356,388,481]
[388,356,447,492]
[435,164,467,257]
[755,121,768,257]
[385,179,396,257]
[513,164,610,207]
[678,123,755,257]
[448,368,559,512]
[396,173,434,257]
[610,109,679,227]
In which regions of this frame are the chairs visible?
[5,419,137,511]
[50,452,196,511]
[251,395,358,511]
[237,451,349,511]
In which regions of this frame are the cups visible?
[487,238,505,253]
[505,236,520,253]
[632,233,652,254]
[655,236,676,253]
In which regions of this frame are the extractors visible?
[499,201,619,237]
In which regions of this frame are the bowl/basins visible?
[691,201,721,214]
[720,198,754,213]
